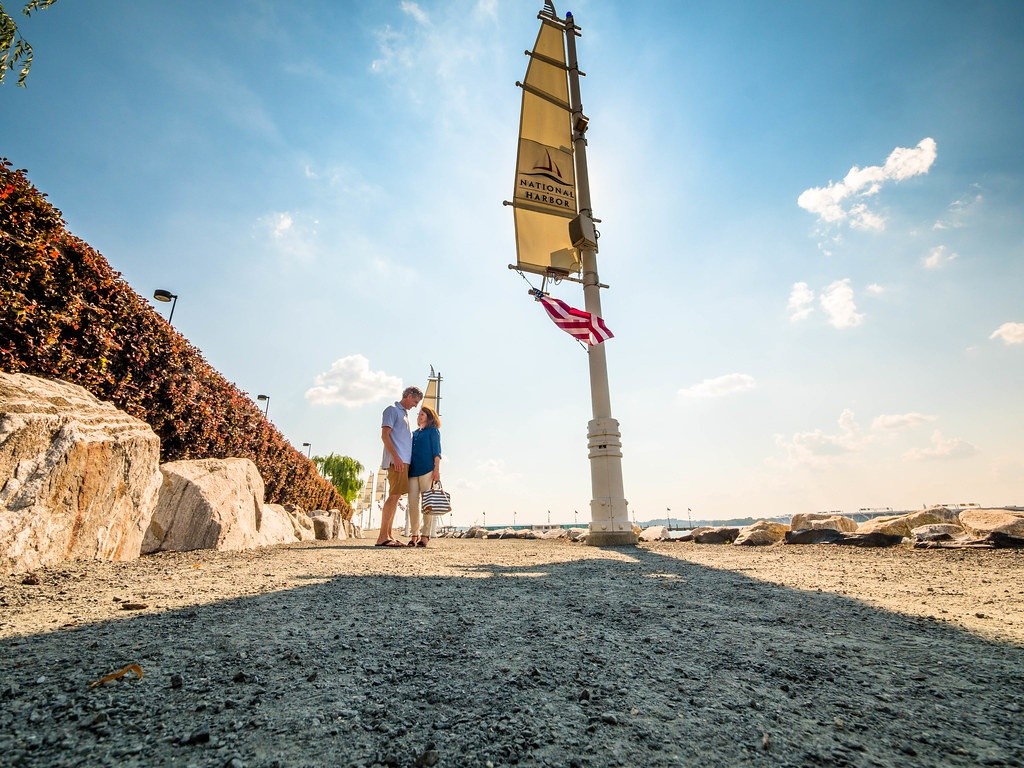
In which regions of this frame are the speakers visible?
[569,213,596,250]
[551,247,580,274]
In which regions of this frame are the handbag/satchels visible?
[421,478,452,516]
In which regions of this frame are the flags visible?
[533,288,614,347]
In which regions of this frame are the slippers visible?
[375,539,406,547]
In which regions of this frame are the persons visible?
[407,407,442,547]
[375,384,423,547]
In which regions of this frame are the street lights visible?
[304,442,311,458]
[154,289,178,324]
[257,395,269,416]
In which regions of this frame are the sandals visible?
[416,535,430,548]
[408,535,420,547]
